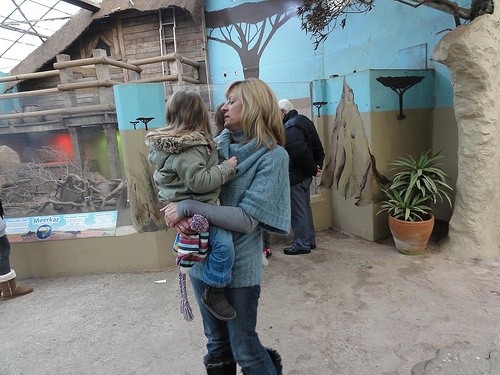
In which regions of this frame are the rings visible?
[180,226,183,230]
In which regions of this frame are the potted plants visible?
[375,149,453,255]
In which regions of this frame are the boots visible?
[203,350,236,375]
[200,283,236,321]
[0,269,33,300]
[242,348,283,375]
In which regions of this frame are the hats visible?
[176,214,209,322]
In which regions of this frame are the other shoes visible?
[263,248,271,257]
[284,244,316,254]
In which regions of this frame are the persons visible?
[189,78,291,375]
[278,99,326,255]
[145,90,237,321]
[0,199,33,301]
[213,103,273,266]
